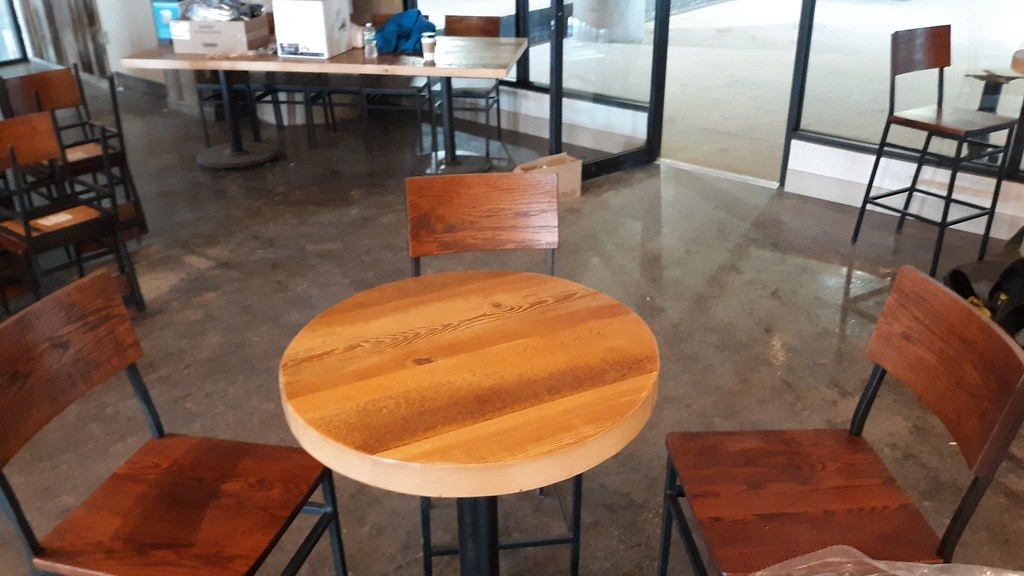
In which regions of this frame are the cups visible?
[421,32,436,38]
[421,38,436,61]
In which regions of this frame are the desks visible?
[122,34,527,173]
[277,274,661,576]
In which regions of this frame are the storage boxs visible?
[151,0,193,46]
[272,0,354,59]
[170,15,269,56]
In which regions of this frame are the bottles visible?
[362,23,378,59]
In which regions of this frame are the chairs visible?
[850,26,1019,277]
[1,64,348,576]
[430,15,503,158]
[657,263,1024,576]
[404,172,585,576]
